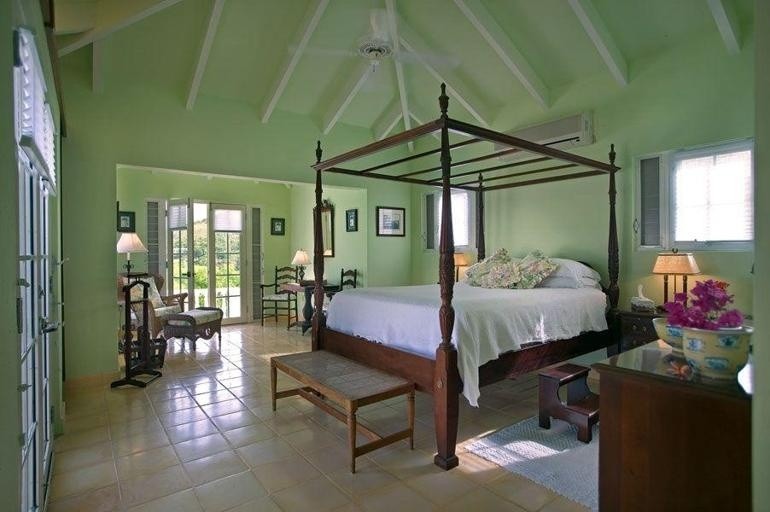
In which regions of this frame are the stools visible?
[161,305,224,352]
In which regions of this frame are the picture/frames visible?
[375,205,406,237]
[346,209,358,232]
[118,211,136,233]
[270,218,285,236]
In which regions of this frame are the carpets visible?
[462,400,602,511]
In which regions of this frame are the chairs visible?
[118,272,189,345]
[326,269,357,302]
[259,266,298,331]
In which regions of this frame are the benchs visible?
[271,351,416,474]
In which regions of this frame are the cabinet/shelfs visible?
[535,362,601,444]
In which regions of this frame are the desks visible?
[281,282,340,336]
[589,338,752,512]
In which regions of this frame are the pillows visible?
[540,277,603,290]
[546,257,599,280]
[464,248,521,292]
[512,250,560,289]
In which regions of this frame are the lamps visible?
[117,232,148,355]
[453,251,469,283]
[651,248,702,309]
[291,250,311,284]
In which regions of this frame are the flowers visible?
[664,280,748,330]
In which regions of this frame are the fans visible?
[284,3,460,94]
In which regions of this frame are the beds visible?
[310,83,621,470]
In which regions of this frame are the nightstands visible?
[618,309,668,354]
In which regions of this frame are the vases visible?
[652,317,683,359]
[683,327,755,380]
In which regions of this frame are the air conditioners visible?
[494,111,595,163]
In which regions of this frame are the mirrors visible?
[311,199,336,258]
